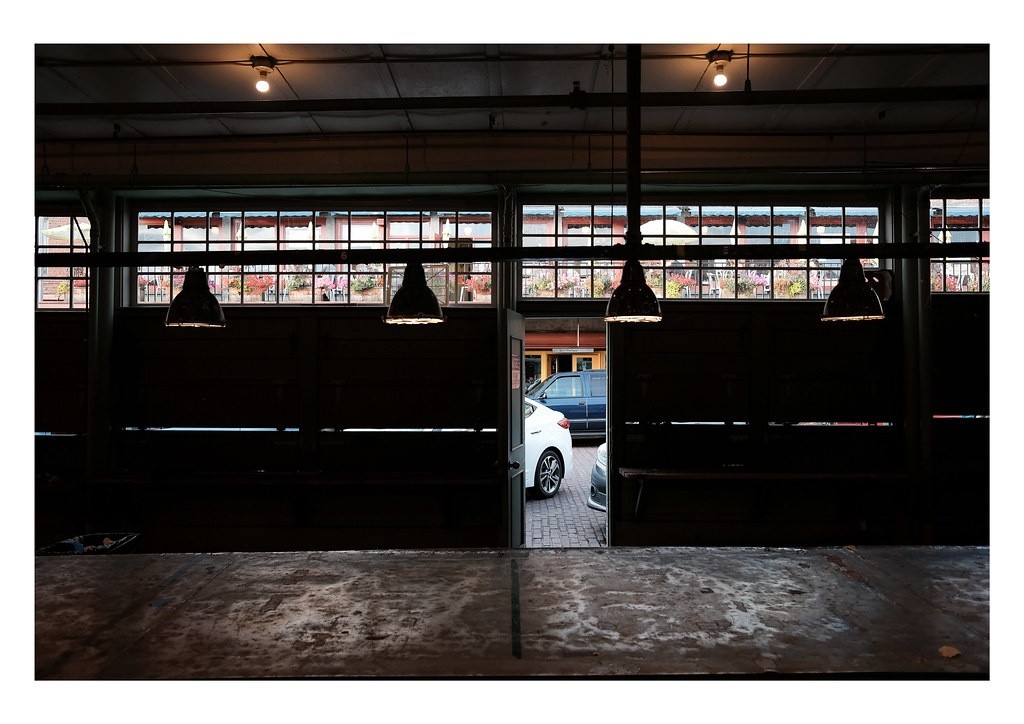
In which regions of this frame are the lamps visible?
[165,266,226,328]
[381,261,447,324]
[604,261,663,323]
[820,257,886,321]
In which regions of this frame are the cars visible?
[523,397,573,497]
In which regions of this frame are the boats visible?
[36,532,141,554]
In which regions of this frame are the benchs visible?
[619,467,909,519]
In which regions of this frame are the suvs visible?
[524,369,607,439]
